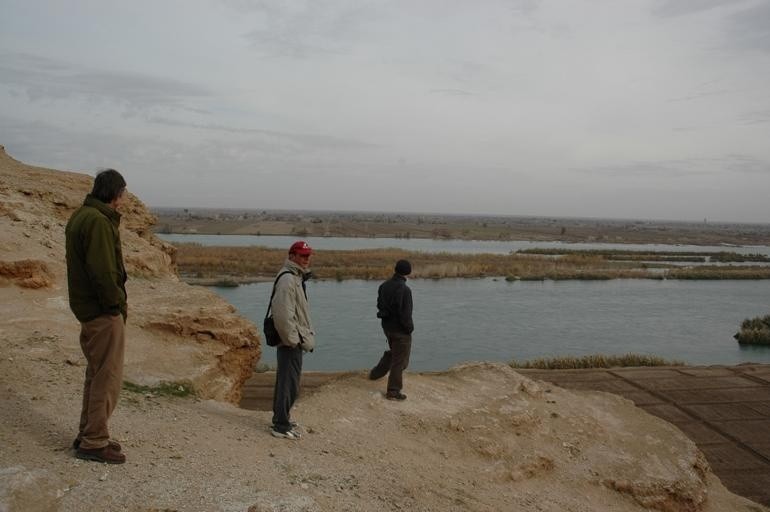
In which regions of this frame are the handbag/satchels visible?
[264,318,281,346]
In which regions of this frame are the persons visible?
[269,240,315,441]
[65,170,129,463]
[369,260,415,401]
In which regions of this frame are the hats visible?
[288,241,313,254]
[396,260,411,275]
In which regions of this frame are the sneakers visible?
[387,392,406,400]
[73,438,126,464]
[270,420,307,440]
